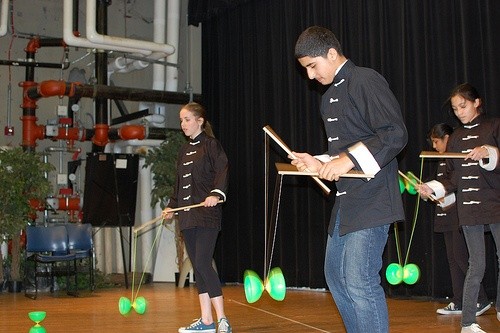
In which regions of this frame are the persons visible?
[430,123,493,316]
[416,87,500,333]
[161,103,233,333]
[288,25,409,332]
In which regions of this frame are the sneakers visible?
[177,318,216,333]
[436,302,462,315]
[216,316,233,333]
[475,302,491,316]
[461,323,487,333]
[496,312,500,321]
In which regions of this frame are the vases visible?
[7,280,22,293]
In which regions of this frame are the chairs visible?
[25,223,94,300]
